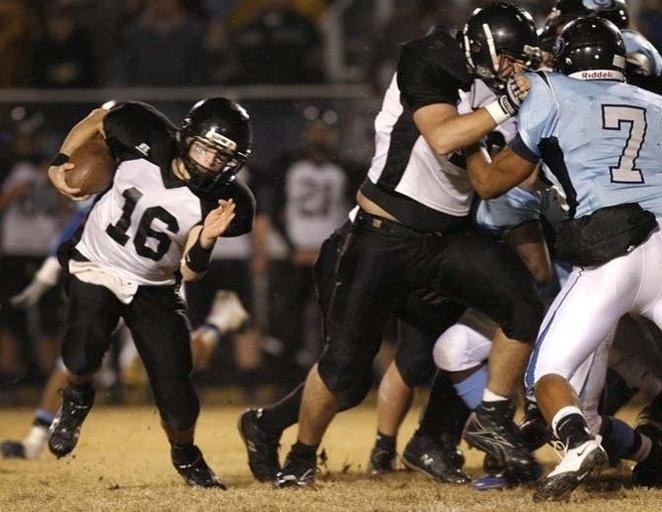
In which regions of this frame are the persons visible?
[0,0,471,408]
[238,0,662,504]
[1,98,255,493]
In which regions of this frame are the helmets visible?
[455,1,629,92]
[177,95,252,188]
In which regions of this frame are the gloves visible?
[11,278,53,308]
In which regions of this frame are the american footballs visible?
[65,139,117,197]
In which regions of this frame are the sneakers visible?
[172,447,225,488]
[1,438,41,459]
[49,390,94,455]
[370,402,662,501]
[237,406,319,487]
